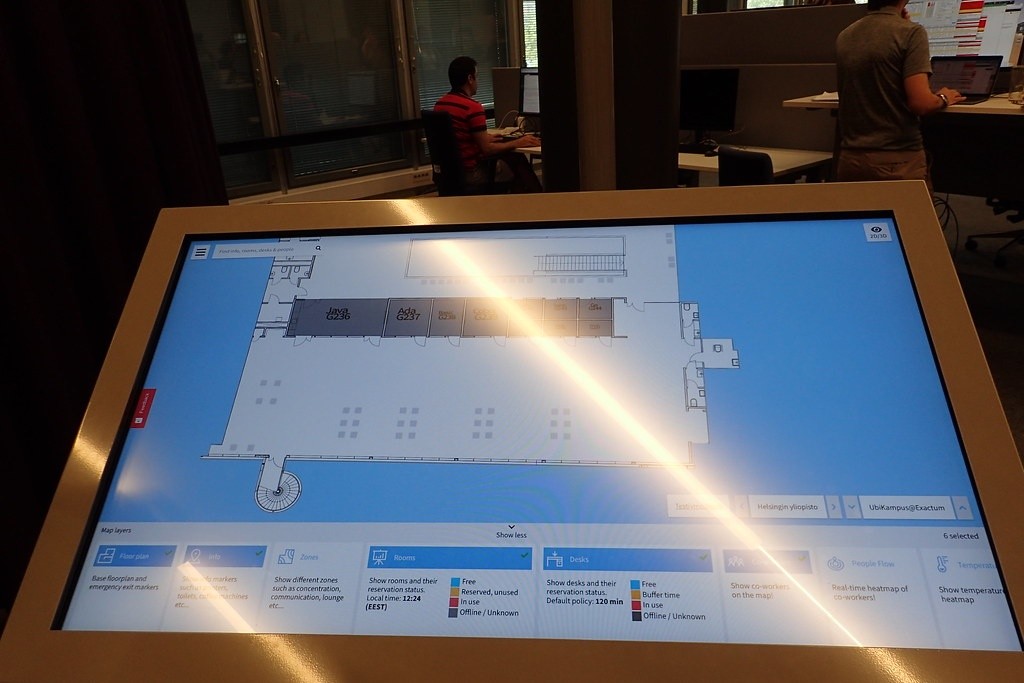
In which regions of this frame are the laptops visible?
[924,56,1003,104]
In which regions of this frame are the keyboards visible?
[501,136,522,142]
[679,144,720,154]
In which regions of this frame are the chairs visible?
[964,120,1024,266]
[718,144,775,186]
[425,114,476,197]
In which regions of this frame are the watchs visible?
[937,94,949,110]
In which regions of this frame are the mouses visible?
[703,150,719,157]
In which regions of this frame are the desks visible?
[678,144,832,186]
[783,91,1024,182]
[423,128,542,168]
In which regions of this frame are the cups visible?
[1008,64,1024,104]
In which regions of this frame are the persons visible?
[293,30,311,48]
[835,0,967,182]
[268,31,287,64]
[433,56,541,195]
[218,40,245,83]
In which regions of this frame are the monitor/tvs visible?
[0,179,1022,682]
[519,70,540,116]
[679,67,740,142]
